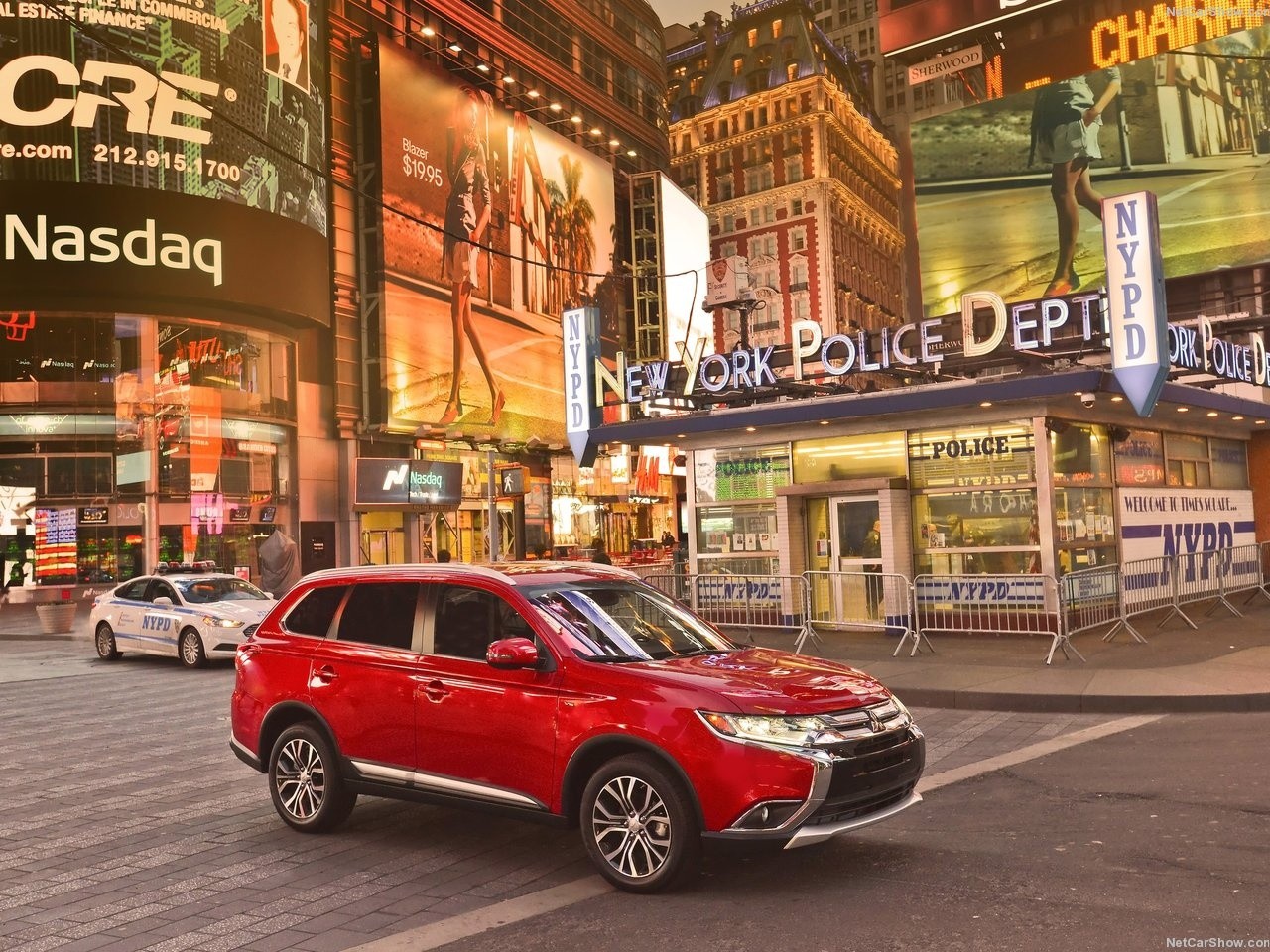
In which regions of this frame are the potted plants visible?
[36,600,78,633]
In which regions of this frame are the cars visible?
[88,560,278,671]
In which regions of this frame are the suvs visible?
[227,555,927,896]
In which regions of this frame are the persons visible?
[437,538,611,566]
[436,89,506,425]
[266,0,308,90]
[1027,63,1121,300]
[862,520,883,619]
[662,531,674,546]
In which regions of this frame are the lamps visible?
[497,87,540,100]
[446,432,464,441]
[583,138,620,149]
[414,423,431,436]
[523,101,561,113]
[419,40,461,55]
[474,434,494,445]
[565,125,603,137]
[449,59,492,72]
[1109,426,1130,444]
[393,25,435,37]
[544,114,583,126]
[601,148,637,158]
[525,436,540,449]
[496,438,515,448]
[472,73,516,86]
[1045,421,1069,441]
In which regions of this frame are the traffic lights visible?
[500,465,531,497]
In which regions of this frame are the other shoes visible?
[867,602,878,618]
